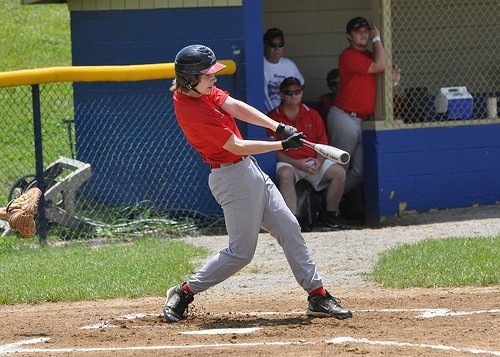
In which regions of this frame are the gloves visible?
[281,132,306,150]
[275,122,299,140]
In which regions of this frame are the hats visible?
[280,77,301,91]
[346,17,369,34]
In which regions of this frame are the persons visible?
[262,17,401,232]
[162,46,353,324]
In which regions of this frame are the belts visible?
[211,154,249,168]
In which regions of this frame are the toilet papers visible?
[487,97,498,120]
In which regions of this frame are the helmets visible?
[174,44,226,74]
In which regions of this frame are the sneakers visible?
[163,281,195,324]
[306,289,353,320]
[321,212,348,228]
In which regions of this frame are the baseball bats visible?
[299,138,350,165]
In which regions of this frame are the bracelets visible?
[372,36,381,43]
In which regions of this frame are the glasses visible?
[353,22,368,29]
[267,41,284,48]
[283,89,302,96]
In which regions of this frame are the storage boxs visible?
[434,86,474,120]
